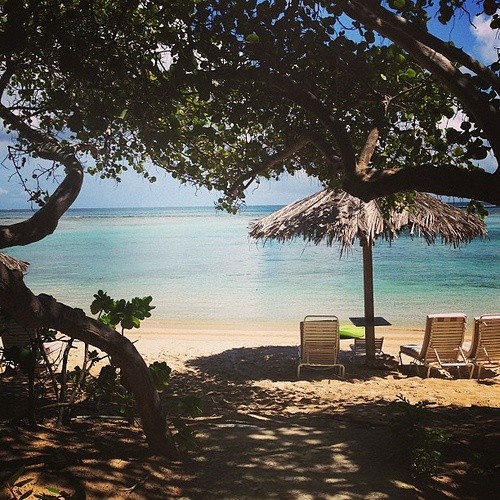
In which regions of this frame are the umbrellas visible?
[247,176,489,379]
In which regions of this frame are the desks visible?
[349,315,391,327]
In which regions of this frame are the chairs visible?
[460,313,500,377]
[350,337,385,365]
[295,315,348,376]
[398,313,476,380]
